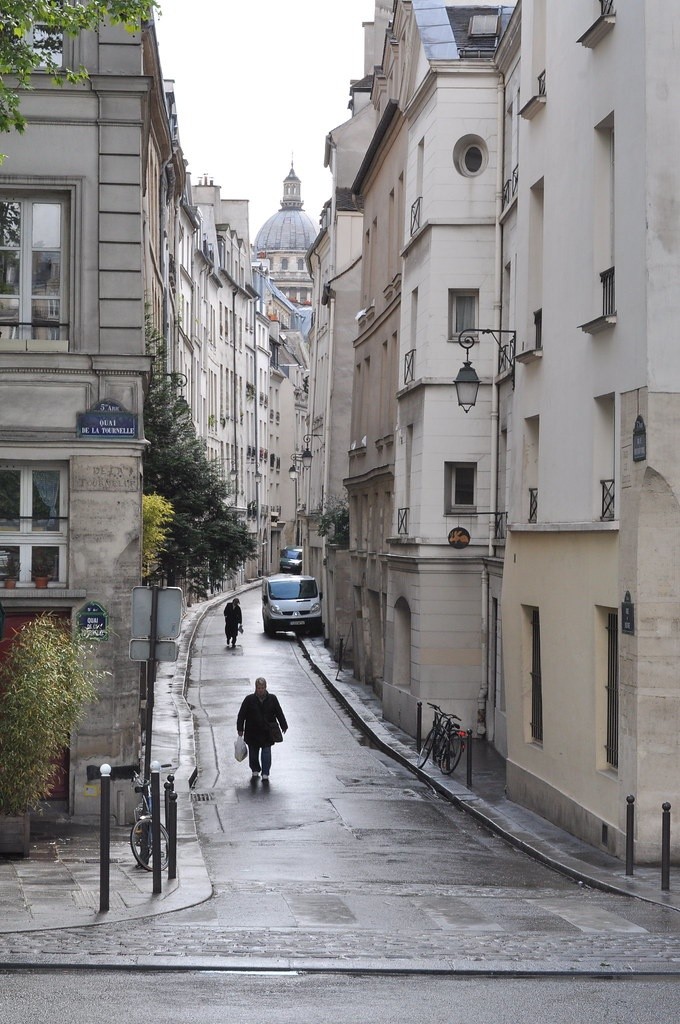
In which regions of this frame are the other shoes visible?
[232,644,236,647]
[227,640,229,645]
[252,771,259,776]
[262,775,268,779]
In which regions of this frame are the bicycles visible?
[417,702,466,775]
[131,770,170,871]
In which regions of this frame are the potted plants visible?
[2,558,23,588]
[30,559,55,588]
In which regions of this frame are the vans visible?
[262,574,323,637]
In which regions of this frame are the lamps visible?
[218,457,238,481]
[245,461,263,484]
[289,453,303,481]
[454,329,516,414]
[302,432,323,471]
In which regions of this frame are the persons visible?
[237,678,288,785]
[224,598,242,647]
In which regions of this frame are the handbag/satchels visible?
[234,735,247,762]
[239,625,243,634]
[268,723,283,743]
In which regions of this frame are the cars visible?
[280,546,302,574]
